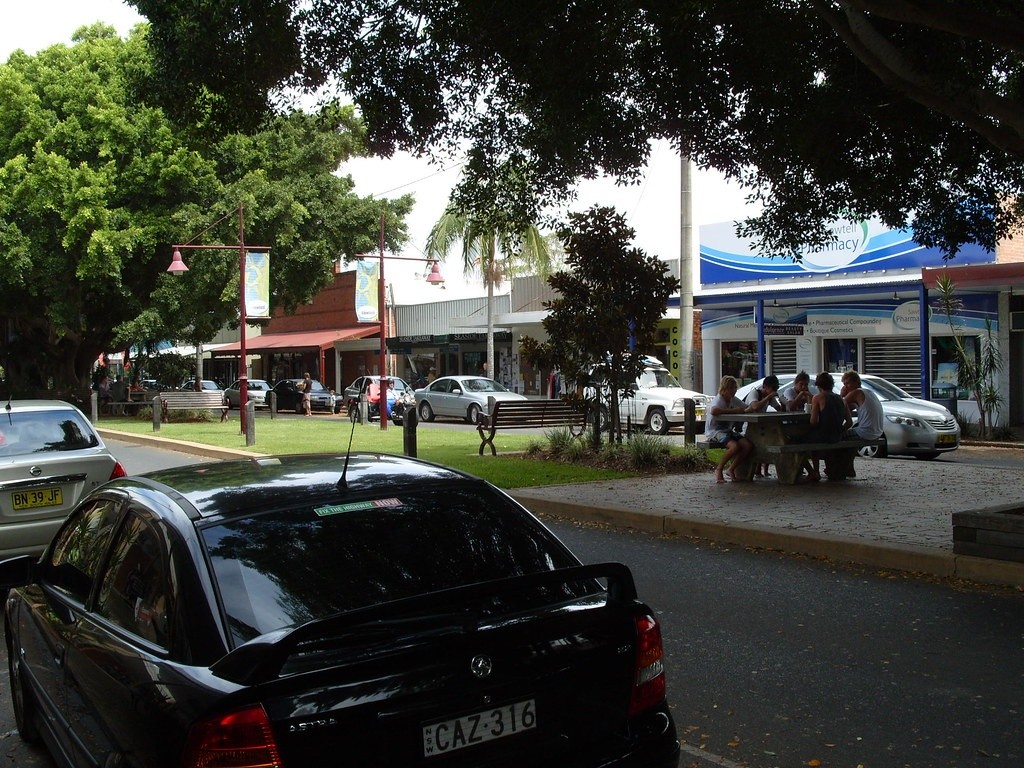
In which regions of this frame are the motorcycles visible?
[349,386,419,427]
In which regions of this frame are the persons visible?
[298,373,313,417]
[781,369,814,480]
[329,390,336,414]
[839,368,885,478]
[386,378,399,418]
[428,366,439,391]
[110,375,132,416]
[119,558,170,615]
[479,362,488,378]
[744,374,785,479]
[704,374,755,484]
[98,374,112,415]
[807,371,854,482]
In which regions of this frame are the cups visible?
[804,404,811,413]
[779,396,789,404]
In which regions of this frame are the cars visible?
[0,398,127,565]
[138,379,157,388]
[732,371,961,460]
[181,380,224,394]
[265,378,344,415]
[222,379,275,409]
[414,376,530,426]
[6,450,682,768]
[344,375,415,404]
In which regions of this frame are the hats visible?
[430,367,437,371]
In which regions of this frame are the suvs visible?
[581,355,717,434]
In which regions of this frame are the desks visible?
[107,392,147,401]
[713,410,858,484]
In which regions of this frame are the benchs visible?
[107,401,153,416]
[767,438,886,453]
[697,442,726,449]
[160,390,230,423]
[476,399,588,456]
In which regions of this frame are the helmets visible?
[387,378,394,384]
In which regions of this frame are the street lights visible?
[167,243,247,437]
[378,255,444,431]
